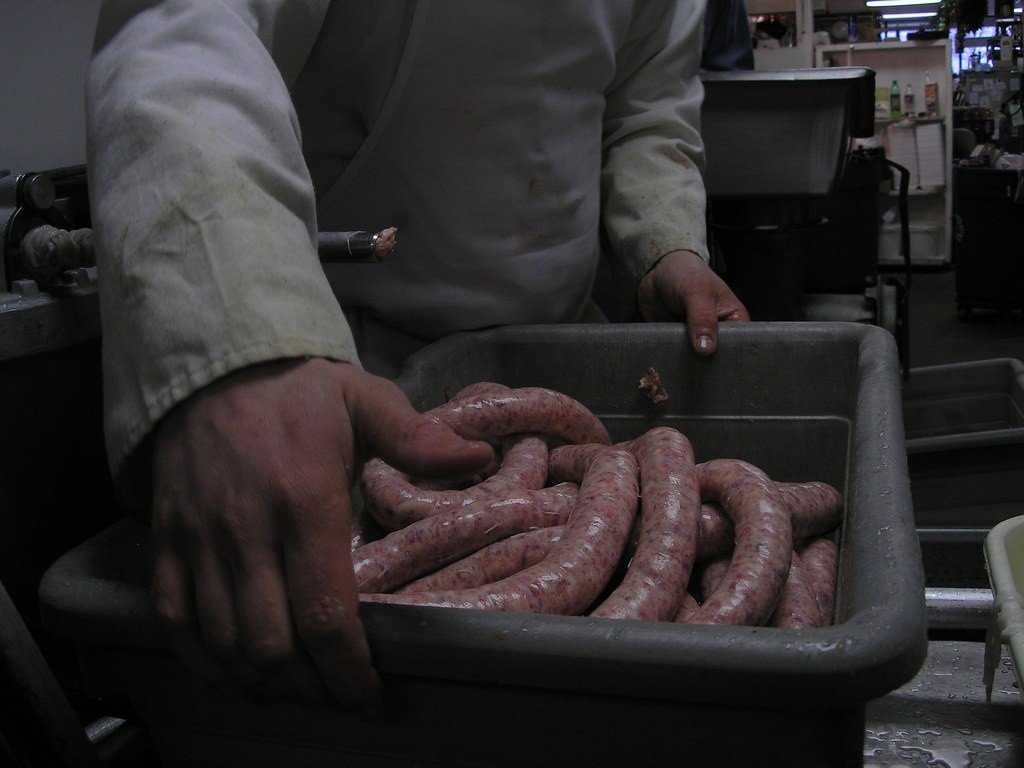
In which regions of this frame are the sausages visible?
[352,380,845,633]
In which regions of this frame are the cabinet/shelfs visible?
[816,38,956,266]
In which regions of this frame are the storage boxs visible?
[702,69,878,197]
[899,356,1023,690]
[38,320,926,767]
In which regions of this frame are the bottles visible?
[975,107,982,130]
[905,84,914,116]
[891,80,901,118]
[925,70,937,112]
[983,107,993,134]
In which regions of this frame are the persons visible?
[85,0,748,720]
